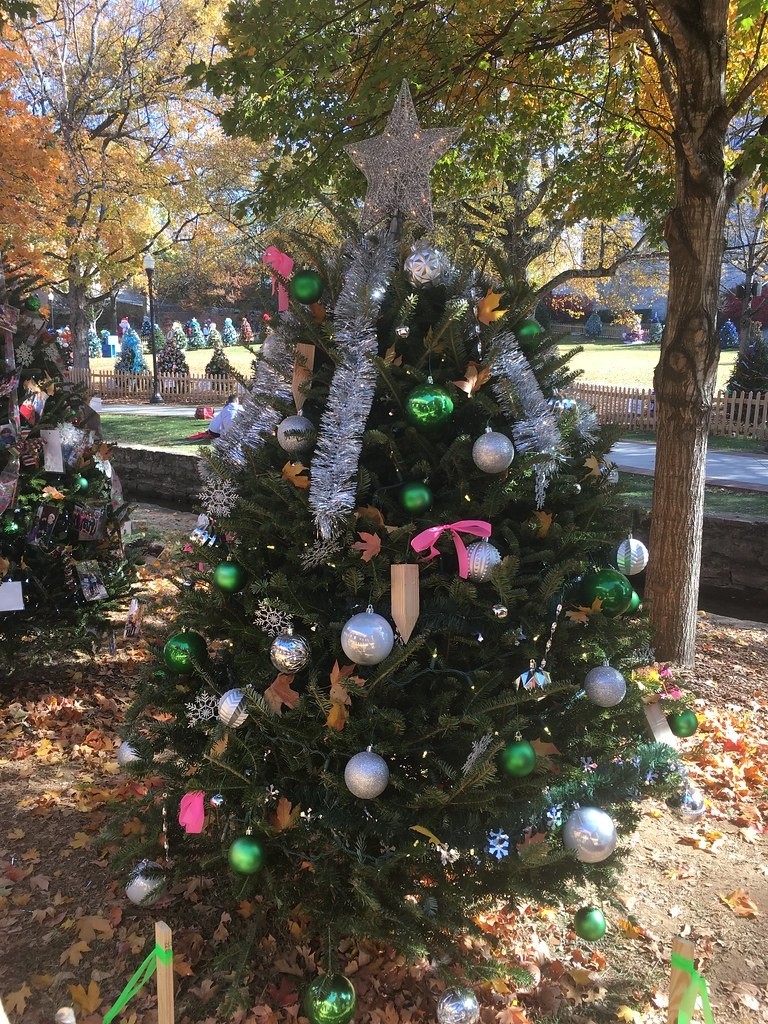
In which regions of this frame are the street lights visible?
[142,252,164,402]
[48,293,55,329]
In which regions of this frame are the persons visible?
[208,394,244,436]
